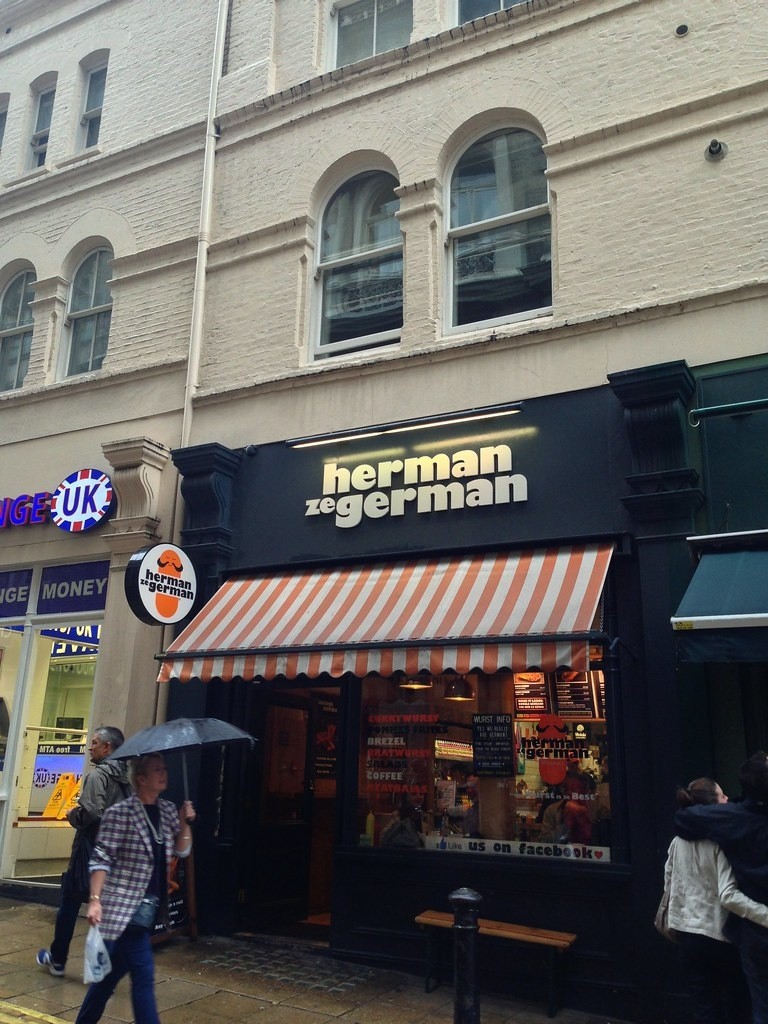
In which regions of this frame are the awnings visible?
[150,544,617,682]
[669,536,767,632]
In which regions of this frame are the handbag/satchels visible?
[654,893,688,944]
[61,809,95,901]
[129,893,159,929]
[83,923,112,985]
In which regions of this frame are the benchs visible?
[414,911,577,1018]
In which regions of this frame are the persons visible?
[667,748,768,1024]
[72,750,200,1024]
[34,723,138,981]
[648,775,768,1024]
[367,755,609,852]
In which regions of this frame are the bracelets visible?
[86,894,100,901]
[177,835,192,841]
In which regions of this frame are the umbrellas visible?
[101,716,259,825]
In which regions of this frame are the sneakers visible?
[36,948,64,976]
